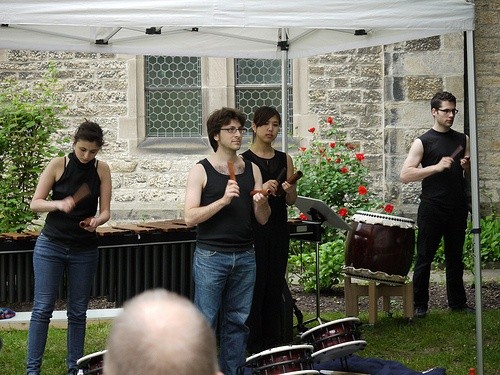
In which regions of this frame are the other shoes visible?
[452,306,476,314]
[414,308,426,319]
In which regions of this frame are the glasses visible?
[436,108,458,114]
[219,126,247,134]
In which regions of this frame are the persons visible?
[102,288,224,375]
[399,91,474,318]
[185,107,271,375]
[239,107,298,354]
[25,118,112,375]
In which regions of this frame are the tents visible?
[0,0,485,375]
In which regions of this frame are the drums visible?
[301,317,368,362]
[339,211,416,286]
[77,350,107,375]
[246,345,320,375]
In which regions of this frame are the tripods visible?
[293,194,353,328]
